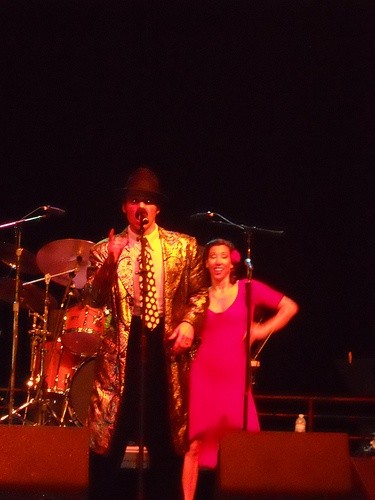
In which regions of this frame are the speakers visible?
[348,457,375,500]
[0,426,103,500]
[215,431,352,500]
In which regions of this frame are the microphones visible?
[135,208,148,224]
[42,205,66,215]
[188,213,214,222]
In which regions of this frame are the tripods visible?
[0,267,77,426]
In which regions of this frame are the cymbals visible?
[35,238,97,290]
[0,277,58,313]
[0,241,42,275]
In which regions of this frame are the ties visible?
[137,237,160,332]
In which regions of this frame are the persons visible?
[79,168,211,500]
[178,238,301,500]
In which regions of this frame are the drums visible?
[57,303,112,358]
[66,354,102,427]
[47,308,68,341]
[30,340,79,401]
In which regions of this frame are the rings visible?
[186,342,190,345]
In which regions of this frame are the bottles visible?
[295,413,308,433]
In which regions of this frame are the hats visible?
[119,168,160,195]
